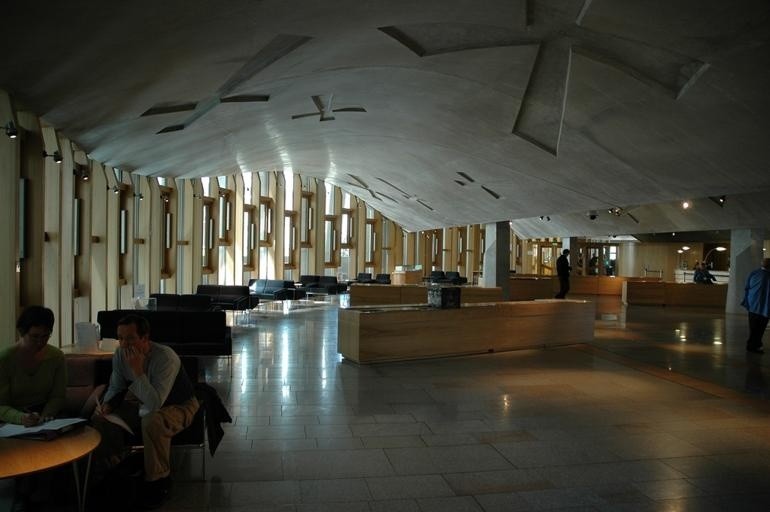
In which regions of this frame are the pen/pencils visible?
[95,391,106,417]
[24,407,39,421]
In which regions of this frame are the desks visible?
[0,424,102,512]
[58,342,118,374]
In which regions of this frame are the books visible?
[14,422,88,440]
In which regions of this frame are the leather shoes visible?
[138,488,172,509]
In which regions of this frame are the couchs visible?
[246,274,391,311]
[91,284,259,377]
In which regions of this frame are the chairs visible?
[423,269,467,286]
[98,357,209,479]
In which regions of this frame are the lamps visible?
[705,245,727,269]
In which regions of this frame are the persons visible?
[742,257,770,353]
[88,313,202,509]
[554,249,574,300]
[0,302,68,429]
[577,253,599,275]
[693,262,701,284]
[700,262,716,284]
